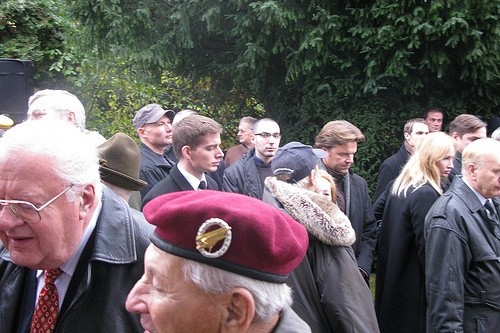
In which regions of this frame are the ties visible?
[485,201,497,221]
[28,268,63,333]
[199,181,207,190]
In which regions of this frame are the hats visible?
[97,132,148,192]
[142,189,309,283]
[132,104,175,132]
[271,142,328,185]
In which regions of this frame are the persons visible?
[139,110,224,213]
[132,102,176,201]
[0,116,156,333]
[447,113,487,184]
[27,89,86,128]
[375,133,458,333]
[376,118,429,206]
[263,141,380,333]
[222,116,282,201]
[424,105,443,132]
[96,132,148,201]
[424,128,500,333]
[314,119,377,289]
[125,190,311,333]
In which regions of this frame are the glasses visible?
[0,183,75,224]
[254,132,281,139]
